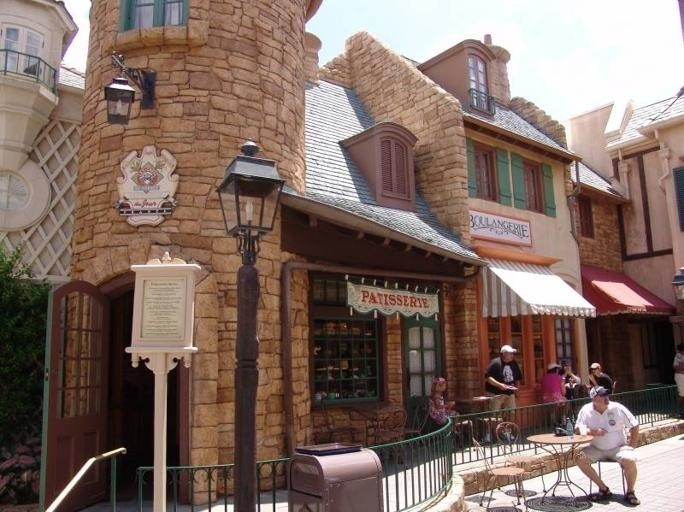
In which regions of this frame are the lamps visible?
[101,48,160,125]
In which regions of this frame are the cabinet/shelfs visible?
[313,315,384,408]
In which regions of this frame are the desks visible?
[527,433,594,508]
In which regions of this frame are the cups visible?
[579,425,587,437]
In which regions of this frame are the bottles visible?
[566,417,575,440]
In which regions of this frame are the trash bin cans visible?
[287,442,384,512]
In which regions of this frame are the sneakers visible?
[484,430,514,443]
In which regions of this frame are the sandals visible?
[589,485,615,501]
[625,491,642,505]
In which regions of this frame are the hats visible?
[589,362,604,370]
[589,385,610,397]
[547,358,569,370]
[499,343,517,354]
[434,376,448,384]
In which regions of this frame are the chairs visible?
[468,433,529,512]
[316,394,517,465]
[542,378,619,432]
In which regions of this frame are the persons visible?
[545,363,612,423]
[575,387,642,506]
[674,342,683,419]
[429,378,459,434]
[485,345,522,439]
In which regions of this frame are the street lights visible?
[212,137,291,512]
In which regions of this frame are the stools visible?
[589,456,628,497]
